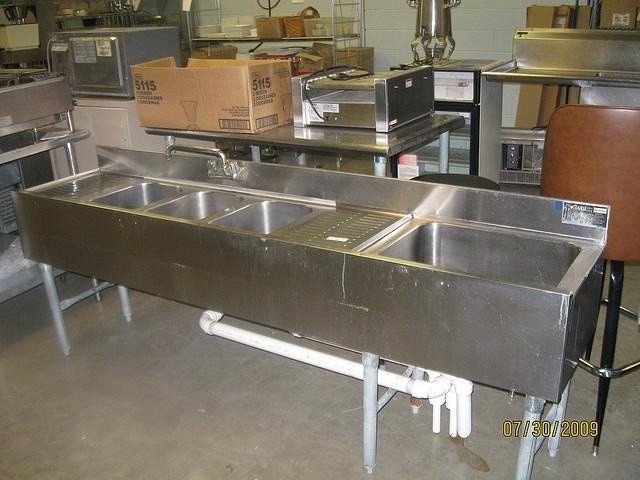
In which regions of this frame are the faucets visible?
[165,143,226,170]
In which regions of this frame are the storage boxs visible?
[295,42,335,73]
[335,46,374,75]
[128,55,293,134]
[600,1,635,30]
[305,18,354,36]
[256,16,285,38]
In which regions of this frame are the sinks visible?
[363,218,604,296]
[90,183,183,209]
[145,190,242,220]
[209,199,312,235]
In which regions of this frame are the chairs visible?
[541,105,639,456]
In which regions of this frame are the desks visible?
[145,116,465,176]
